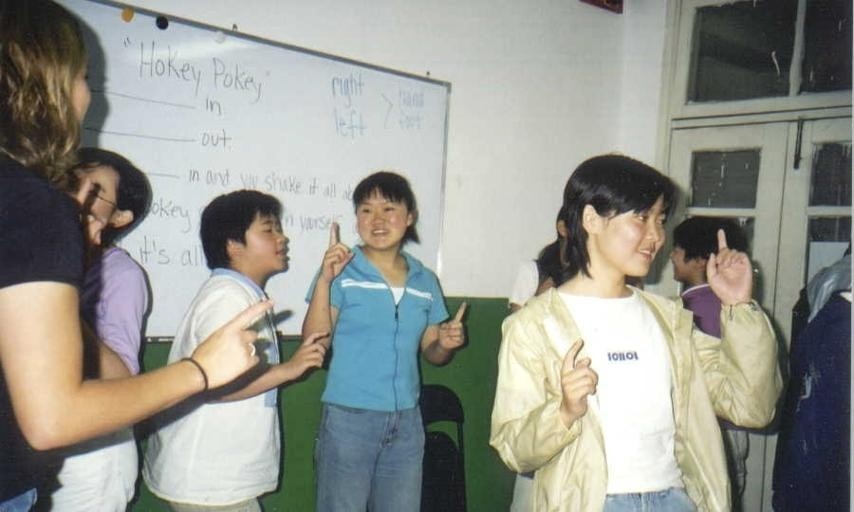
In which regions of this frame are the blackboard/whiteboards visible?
[60,4,452,345]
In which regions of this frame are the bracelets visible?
[181,357,208,394]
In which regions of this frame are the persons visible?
[669,215,749,512]
[507,207,645,511]
[301,170,469,511]
[0,0,277,512]
[487,152,783,512]
[771,245,852,511]
[141,187,330,512]
[32,146,148,512]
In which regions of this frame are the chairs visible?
[418,385,465,512]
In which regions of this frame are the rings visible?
[248,343,256,357]
